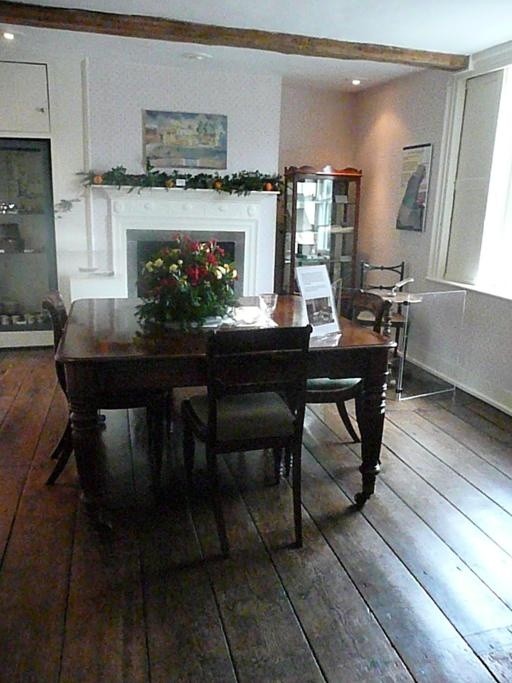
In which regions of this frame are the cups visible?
[259,293,278,312]
[0,312,45,325]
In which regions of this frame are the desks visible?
[53,294,397,536]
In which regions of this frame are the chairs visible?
[355,259,411,393]
[284,291,393,477]
[41,288,173,492]
[180,324,312,558]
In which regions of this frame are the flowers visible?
[76,162,294,195]
[130,227,240,352]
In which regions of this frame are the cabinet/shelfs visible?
[280,165,363,321]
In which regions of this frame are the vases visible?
[144,317,203,339]
